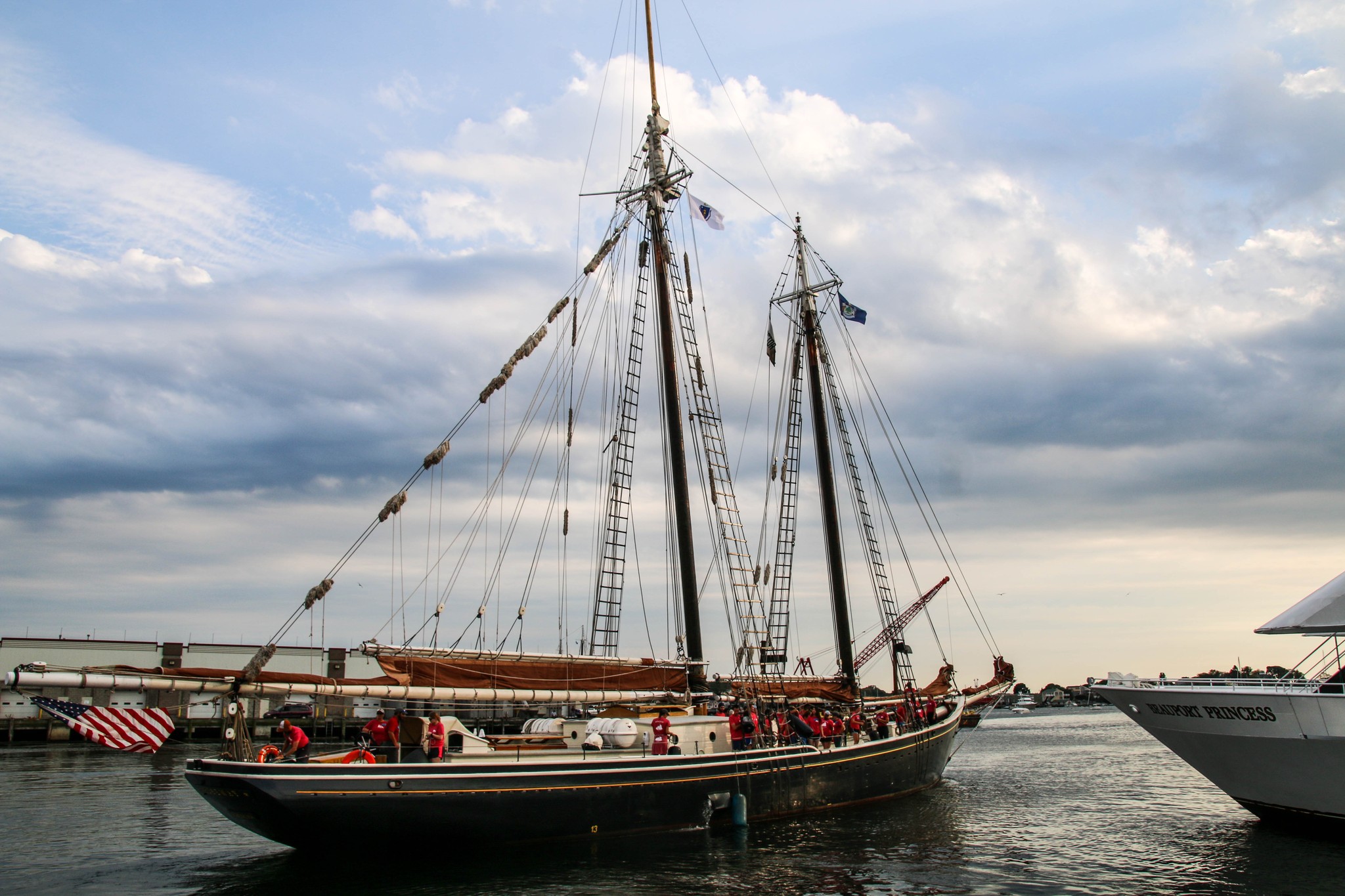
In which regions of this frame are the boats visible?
[1014,692,1036,710]
[1064,703,1078,708]
[1089,568,1345,845]
[1012,708,1030,714]
[1091,704,1101,709]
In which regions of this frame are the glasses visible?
[400,710,406,712]
[428,717,435,720]
[376,713,383,715]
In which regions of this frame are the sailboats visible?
[179,0,1016,861]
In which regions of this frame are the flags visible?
[688,193,725,232]
[837,290,867,325]
[767,309,776,367]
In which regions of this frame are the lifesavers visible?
[257,745,281,763]
[340,750,376,764]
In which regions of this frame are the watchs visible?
[282,753,286,758]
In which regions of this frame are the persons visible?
[362,707,390,763]
[717,692,938,751]
[421,711,445,763]
[386,708,407,763]
[903,682,916,697]
[275,721,310,763]
[650,709,675,755]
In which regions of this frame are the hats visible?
[276,719,291,732]
[830,712,839,716]
[376,708,385,714]
[906,682,912,687]
[659,709,670,716]
[394,708,408,714]
[824,710,832,715]
